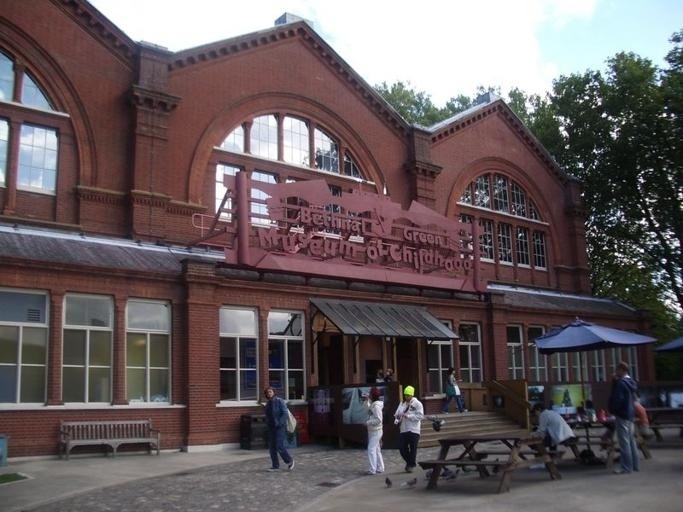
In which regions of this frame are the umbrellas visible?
[653,337,683,352]
[533,316,658,450]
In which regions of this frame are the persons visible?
[393,386,424,472]
[440,368,468,414]
[265,387,294,471]
[530,404,576,470]
[362,388,384,475]
[608,362,640,473]
[633,397,650,432]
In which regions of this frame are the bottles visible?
[289,391,304,400]
[577,408,607,422]
[551,402,568,415]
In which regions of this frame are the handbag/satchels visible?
[285,407,297,434]
[446,383,456,397]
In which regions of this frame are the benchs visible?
[558,435,653,446]
[645,408,683,441]
[417,448,566,468]
[57,417,160,461]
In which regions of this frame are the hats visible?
[403,385,415,397]
[370,387,380,396]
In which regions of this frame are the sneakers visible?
[613,468,628,474]
[440,411,449,414]
[288,458,295,470]
[266,468,280,472]
[460,409,468,412]
[406,466,412,472]
[529,463,544,470]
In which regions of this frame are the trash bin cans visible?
[240,414,269,450]
[0,434,10,466]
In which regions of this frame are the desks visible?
[568,417,652,464]
[437,433,562,493]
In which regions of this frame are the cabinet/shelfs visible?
[239,414,301,449]
